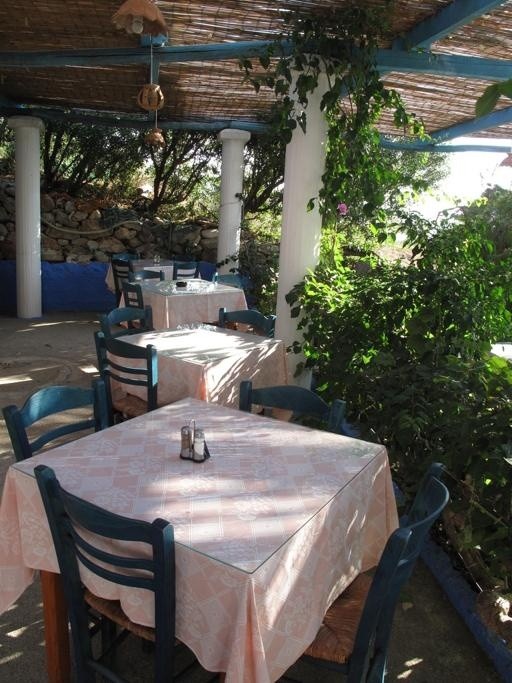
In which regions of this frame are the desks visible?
[0,395,400,683]
[106,320,296,423]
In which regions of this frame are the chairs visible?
[280,462,449,683]
[29,466,197,683]
[1,377,112,463]
[100,253,276,338]
[239,381,346,437]
[94,331,166,428]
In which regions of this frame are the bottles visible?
[180,426,191,459]
[153,255,157,267]
[157,256,160,267]
[193,427,204,462]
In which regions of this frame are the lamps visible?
[137,34,164,112]
[111,0,169,36]
[144,110,166,148]
